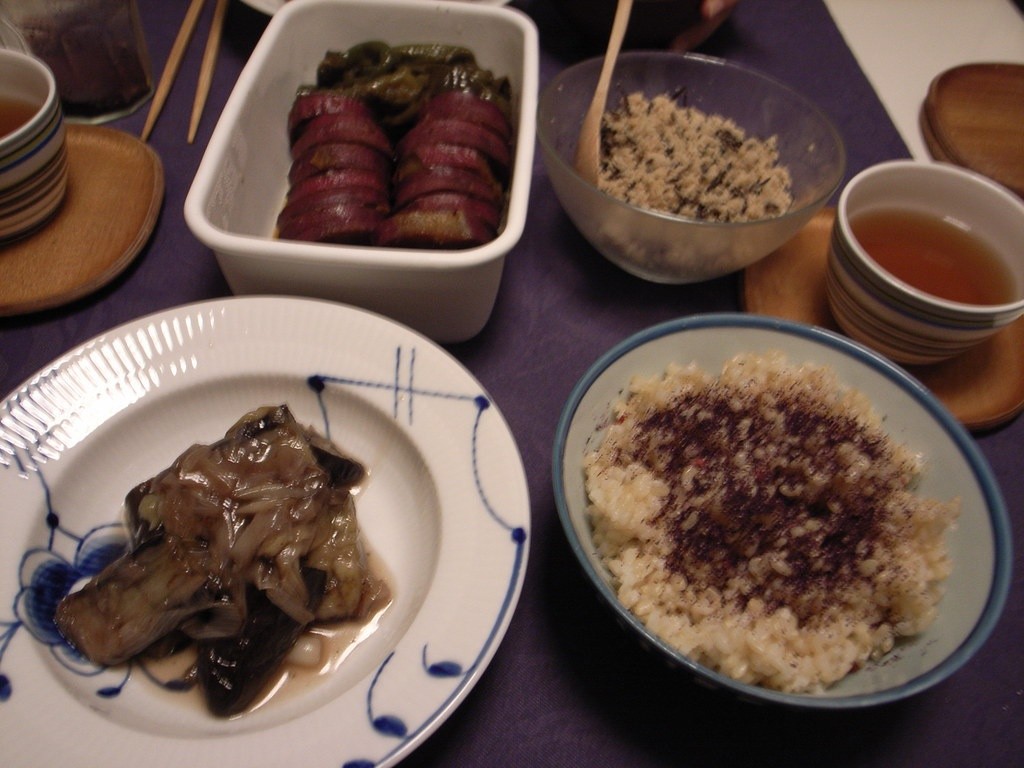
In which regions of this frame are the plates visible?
[744,206,1024,434]
[928,62,1024,194]
[0,292,532,768]
[239,1,519,21]
[0,120,165,316]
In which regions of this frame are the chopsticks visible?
[138,0,233,143]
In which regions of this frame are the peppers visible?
[293,43,511,112]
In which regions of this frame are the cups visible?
[0,43,78,246]
[0,1,154,124]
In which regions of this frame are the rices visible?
[583,352,963,694]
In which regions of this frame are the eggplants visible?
[56,405,390,717]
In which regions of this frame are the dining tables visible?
[0,0,1024,768]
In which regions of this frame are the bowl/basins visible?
[182,1,543,345]
[549,314,1016,715]
[537,50,847,286]
[826,157,1024,365]
